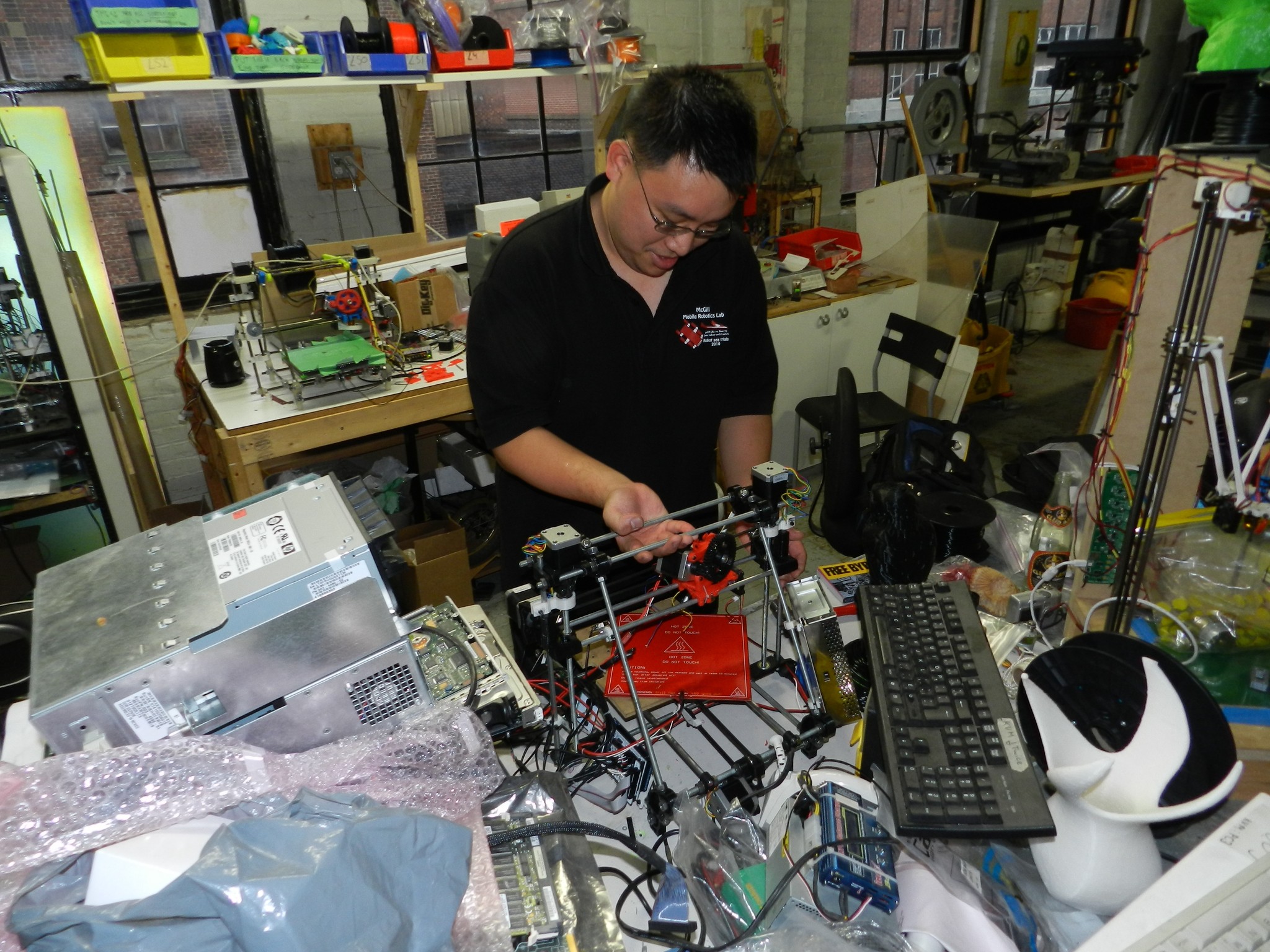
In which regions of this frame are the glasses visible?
[623,137,732,240]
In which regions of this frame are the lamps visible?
[943,46,984,133]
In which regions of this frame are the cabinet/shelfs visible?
[0,146,140,579]
[754,271,921,470]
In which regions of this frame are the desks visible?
[754,185,825,241]
[179,316,473,514]
[957,164,1158,298]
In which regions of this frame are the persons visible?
[1185,0,1270,144]
[466,60,808,614]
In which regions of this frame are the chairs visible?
[790,309,955,503]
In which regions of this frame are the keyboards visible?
[861,579,1057,839]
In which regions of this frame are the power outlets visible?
[330,154,359,180]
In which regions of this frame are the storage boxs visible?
[203,27,327,76]
[320,26,432,76]
[774,224,861,269]
[67,0,202,32]
[387,511,497,611]
[383,270,460,333]
[72,32,213,85]
[432,29,517,72]
[251,231,426,327]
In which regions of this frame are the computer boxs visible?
[28,471,432,754]
